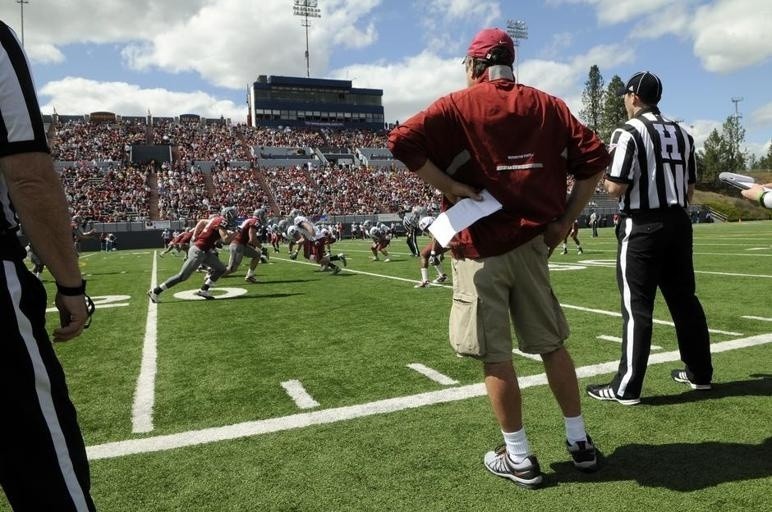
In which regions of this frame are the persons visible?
[690,202,718,226]
[16,115,447,304]
[585,69,713,405]
[389,27,611,488]
[742,181,772,210]
[0,17,96,512]
[559,177,617,256]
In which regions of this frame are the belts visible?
[624,208,685,216]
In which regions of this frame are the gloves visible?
[261,247,267,251]
[409,233,411,236]
[282,232,286,237]
[405,232,407,234]
[315,241,320,245]
[430,256,436,265]
[236,226,241,231]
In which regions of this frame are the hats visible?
[396,210,405,213]
[615,71,663,104]
[462,28,515,65]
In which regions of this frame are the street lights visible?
[293,0,320,78]
[506,20,528,83]
[732,99,740,153]
[18,1,28,47]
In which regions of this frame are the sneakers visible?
[288,250,290,252]
[147,289,159,303]
[261,259,267,263]
[671,367,713,391]
[432,275,450,284]
[414,281,432,289]
[330,268,342,275]
[289,253,297,259]
[577,249,583,254]
[585,384,643,407]
[245,276,257,284]
[410,254,414,256]
[566,432,599,471]
[196,290,212,299]
[415,255,418,256]
[372,258,379,261]
[273,248,275,253]
[261,258,265,261]
[484,443,545,492]
[384,258,392,263]
[159,252,163,258]
[340,254,347,267]
[561,251,565,253]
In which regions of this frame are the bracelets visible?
[759,191,769,208]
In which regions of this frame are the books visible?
[718,173,771,193]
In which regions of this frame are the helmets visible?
[590,208,595,210]
[287,226,301,238]
[222,206,238,222]
[362,220,371,231]
[278,220,287,231]
[290,208,299,219]
[376,221,381,226]
[267,219,274,224]
[419,216,434,232]
[185,227,191,232]
[255,222,259,226]
[252,207,266,219]
[412,206,427,216]
